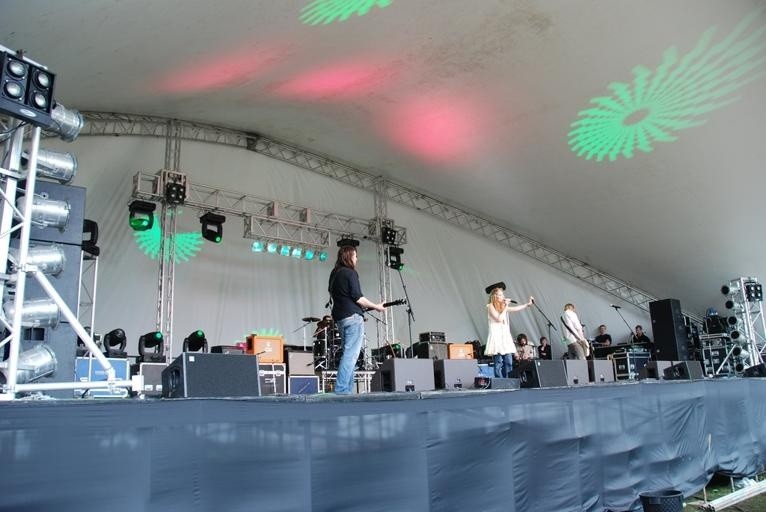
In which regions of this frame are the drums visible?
[314,339,326,358]
[330,328,342,345]
[333,346,364,371]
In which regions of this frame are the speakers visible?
[371,358,435,393]
[588,360,615,383]
[508,360,569,387]
[663,360,705,379]
[648,299,690,361]
[3,178,87,399]
[745,364,766,377]
[672,361,683,367]
[639,361,672,381]
[162,352,261,399]
[565,360,589,385]
[434,359,478,390]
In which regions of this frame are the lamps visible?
[250,238,328,262]
[128,200,156,231]
[183,330,208,354]
[199,212,225,243]
[721,285,750,373]
[0,101,84,383]
[136,331,166,363]
[103,328,127,358]
[385,247,404,270]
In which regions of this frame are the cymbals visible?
[303,318,320,321]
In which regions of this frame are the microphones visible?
[609,304,621,308]
[504,299,517,304]
[388,262,404,267]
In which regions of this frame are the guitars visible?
[362,298,406,321]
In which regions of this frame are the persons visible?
[483,286,536,378]
[513,302,651,388]
[327,244,388,397]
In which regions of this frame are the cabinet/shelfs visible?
[611,352,651,380]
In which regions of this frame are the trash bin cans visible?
[639,490,684,512]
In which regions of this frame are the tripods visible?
[354,333,375,371]
[315,327,338,370]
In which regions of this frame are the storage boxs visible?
[131,363,169,396]
[247,336,319,396]
[75,356,130,398]
[418,332,474,371]
